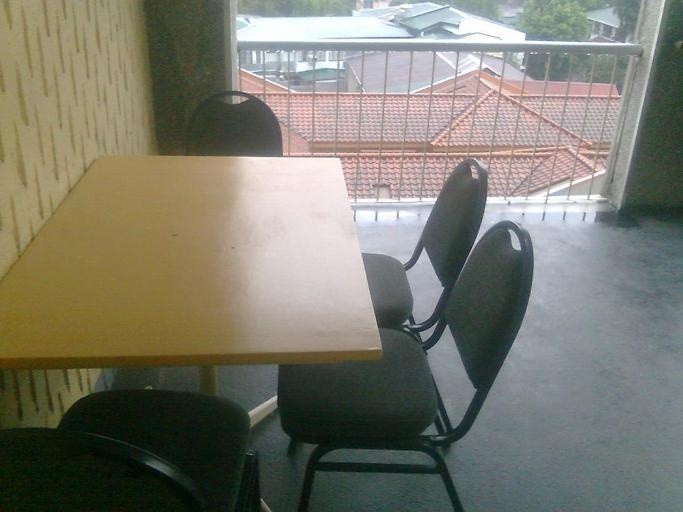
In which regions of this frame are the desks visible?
[1,155,385,512]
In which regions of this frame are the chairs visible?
[359,154,493,444]
[0,389,261,510]
[275,220,535,512]
[188,91,282,156]
